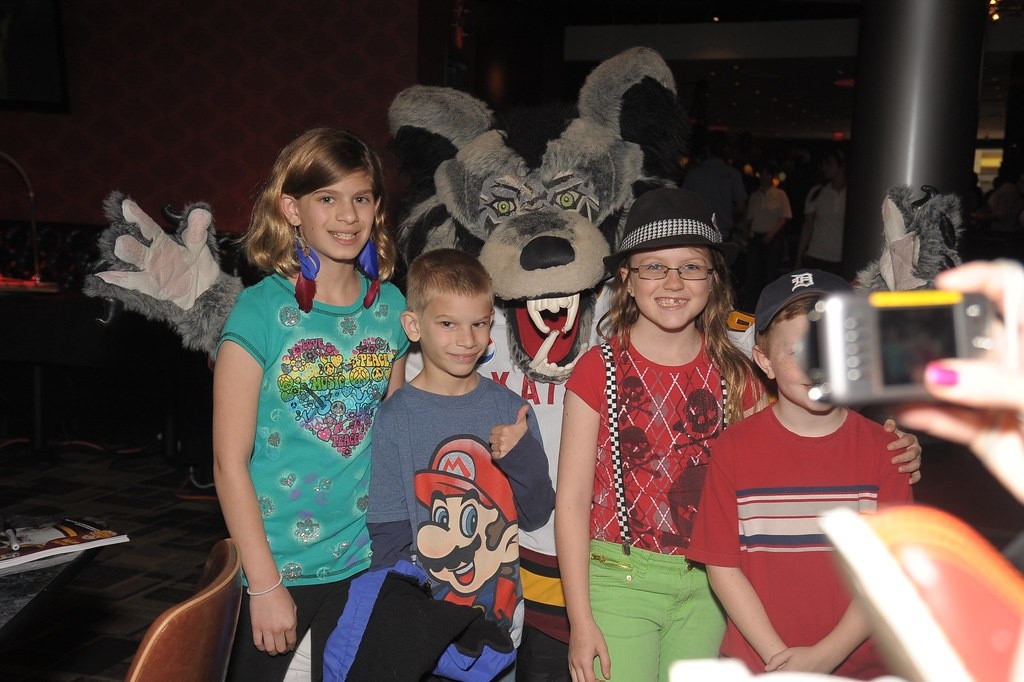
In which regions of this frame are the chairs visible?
[124,536,242,682]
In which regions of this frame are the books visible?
[0,516,131,570]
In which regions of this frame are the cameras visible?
[800,289,993,407]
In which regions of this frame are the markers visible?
[3,520,20,551]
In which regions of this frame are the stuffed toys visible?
[87,48,678,682]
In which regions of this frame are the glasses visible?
[630,263,716,280]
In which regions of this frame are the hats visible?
[755,270,853,335]
[603,188,738,274]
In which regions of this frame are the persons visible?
[212,129,1024,682]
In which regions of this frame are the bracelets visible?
[247,575,283,596]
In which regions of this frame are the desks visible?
[0,494,112,671]
[0,291,212,475]
